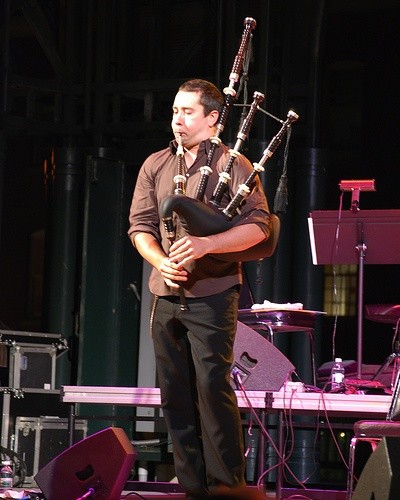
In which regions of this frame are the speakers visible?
[226,321,295,393]
[33,428,136,500]
[352,436,400,500]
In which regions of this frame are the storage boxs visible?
[14,416,87,487]
[0,330,64,388]
[10,346,56,391]
[0,387,71,463]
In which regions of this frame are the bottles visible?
[0,460,14,495]
[331,357,345,393]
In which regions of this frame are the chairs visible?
[345,369,400,500]
[238,278,316,389]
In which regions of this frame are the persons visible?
[128,80,271,500]
